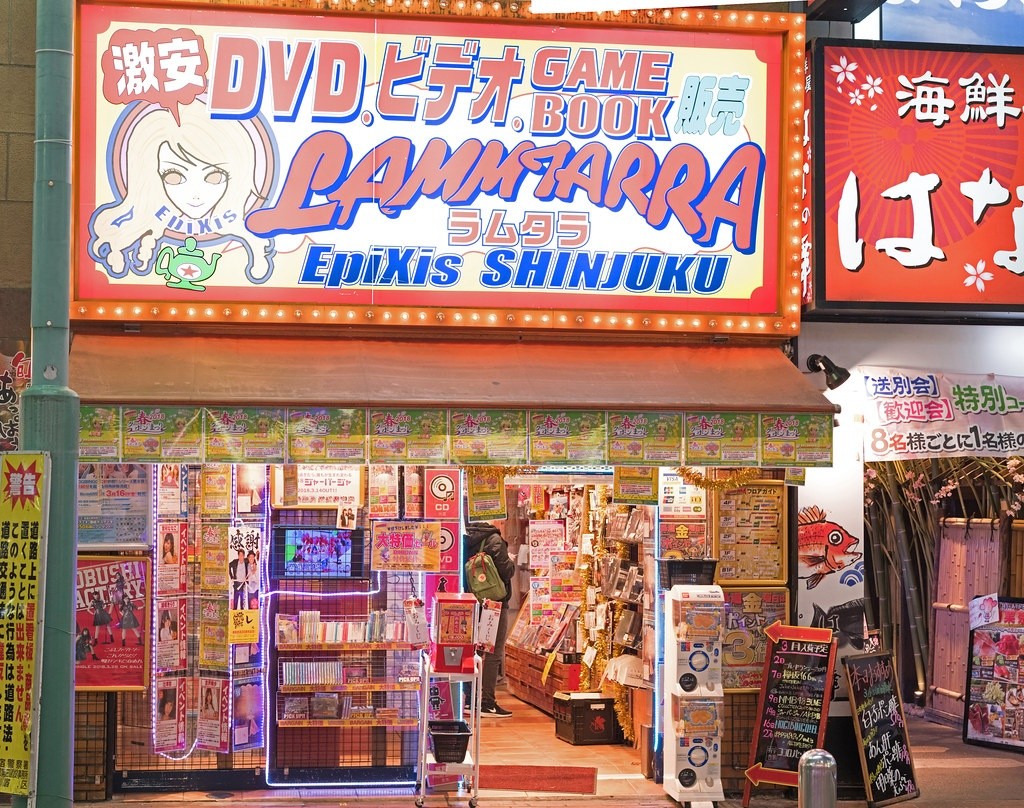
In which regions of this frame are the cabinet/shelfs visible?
[502,484,654,746]
[275,612,483,808]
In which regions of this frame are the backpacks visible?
[465,538,507,606]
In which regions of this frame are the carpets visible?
[473,765,598,793]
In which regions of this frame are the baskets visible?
[657,558,717,591]
[428,720,472,764]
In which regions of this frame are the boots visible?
[138,637,143,645]
[122,639,126,646]
[110,635,114,642]
[93,639,98,647]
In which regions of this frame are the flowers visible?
[931,479,959,505]
[905,472,925,506]
[864,469,876,508]
[1006,459,1024,517]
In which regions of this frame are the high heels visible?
[92,654,99,660]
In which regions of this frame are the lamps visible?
[806,353,851,390]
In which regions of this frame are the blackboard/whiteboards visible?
[745,621,839,786]
[842,651,920,808]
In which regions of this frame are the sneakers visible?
[481,703,512,717]
[463,703,478,716]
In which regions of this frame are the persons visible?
[162,533,177,564]
[118,593,146,646]
[158,693,176,720]
[75,627,99,662]
[229,550,260,611]
[87,591,115,643]
[159,610,175,640]
[462,516,516,718]
[201,688,215,719]
[108,572,125,614]
[340,509,355,527]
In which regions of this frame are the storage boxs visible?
[552,690,617,746]
[433,594,478,674]
[655,557,719,591]
[557,651,585,664]
[426,721,473,794]
